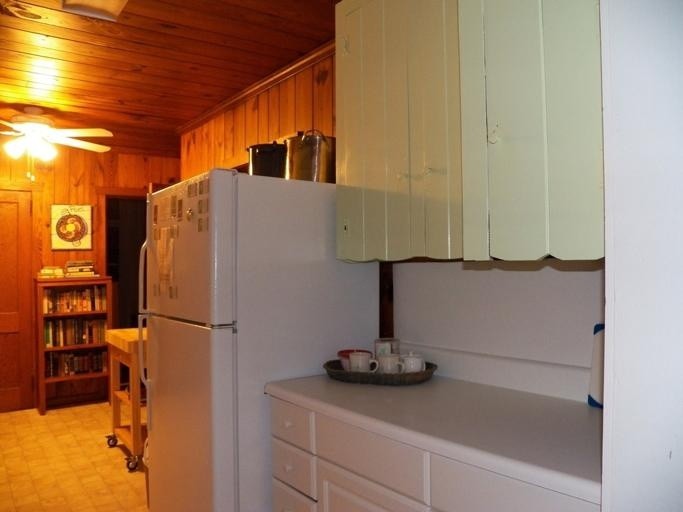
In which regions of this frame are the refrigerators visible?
[139,168,379,511]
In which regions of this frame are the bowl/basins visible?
[338,350,374,371]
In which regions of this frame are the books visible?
[37,260,109,377]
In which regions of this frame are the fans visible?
[0,119,113,154]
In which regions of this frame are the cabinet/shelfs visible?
[269,391,317,512]
[458,1,605,262]
[334,1,462,261]
[105,327,148,471]
[33,276,113,415]
[317,413,601,512]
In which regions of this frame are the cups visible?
[375,337,401,357]
[348,351,378,373]
[402,352,426,374]
[376,353,404,375]
